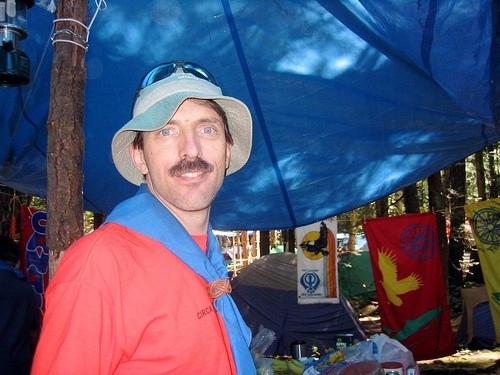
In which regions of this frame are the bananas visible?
[271,358,305,375]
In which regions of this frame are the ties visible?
[99,183,257,375]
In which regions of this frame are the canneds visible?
[334,334,354,351]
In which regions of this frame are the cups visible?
[290,340,306,359]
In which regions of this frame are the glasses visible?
[131,60,217,120]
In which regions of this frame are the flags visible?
[362,211,458,363]
[19,206,47,322]
[464,197,499,346]
[295,218,339,306]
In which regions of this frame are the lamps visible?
[0,0,35,87]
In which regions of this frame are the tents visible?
[227,252,368,358]
[335,251,380,314]
[0,2,500,285]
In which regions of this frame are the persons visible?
[0,235,42,375]
[30,60,261,375]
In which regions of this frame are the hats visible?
[110,67,253,185]
